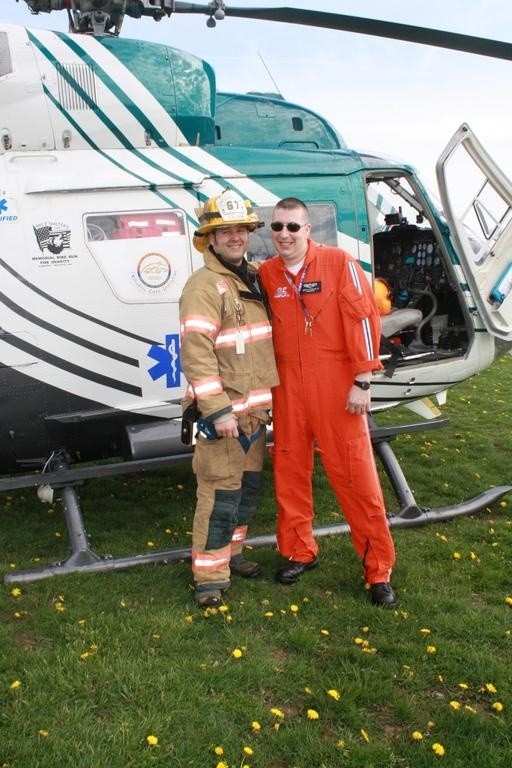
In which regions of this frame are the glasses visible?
[271,222,308,232]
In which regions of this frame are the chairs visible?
[381,307,426,353]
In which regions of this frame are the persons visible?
[181,202,326,606]
[257,197,395,606]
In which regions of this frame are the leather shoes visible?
[372,583,396,608]
[275,555,320,584]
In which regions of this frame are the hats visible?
[193,196,265,253]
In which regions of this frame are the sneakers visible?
[230,559,263,578]
[194,590,222,609]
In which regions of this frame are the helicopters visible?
[0,0,511,586]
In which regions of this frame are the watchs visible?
[354,380,371,390]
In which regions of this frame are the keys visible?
[305,321,313,336]
